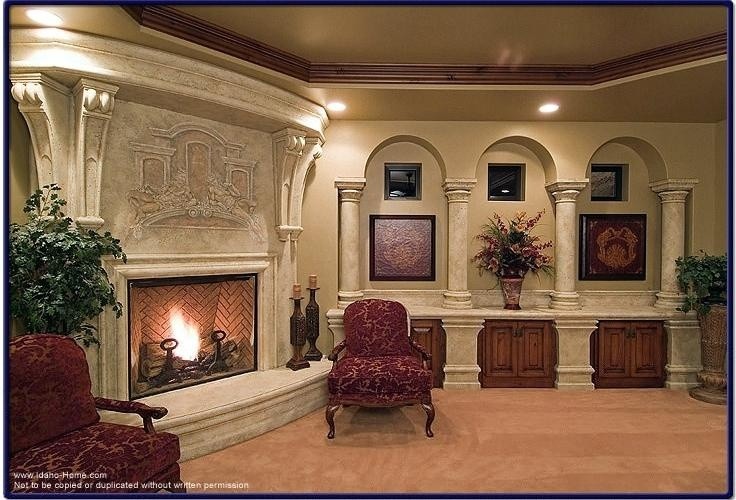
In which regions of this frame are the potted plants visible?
[672,248,728,406]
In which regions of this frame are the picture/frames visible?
[578,213,647,280]
[368,214,437,282]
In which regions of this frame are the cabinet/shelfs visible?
[591,321,669,389]
[478,320,557,388]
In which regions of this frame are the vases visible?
[499,276,525,310]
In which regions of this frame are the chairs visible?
[325,298,436,438]
[8,333,187,494]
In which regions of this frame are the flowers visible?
[471,207,556,291]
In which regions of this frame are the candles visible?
[293,274,317,297]
